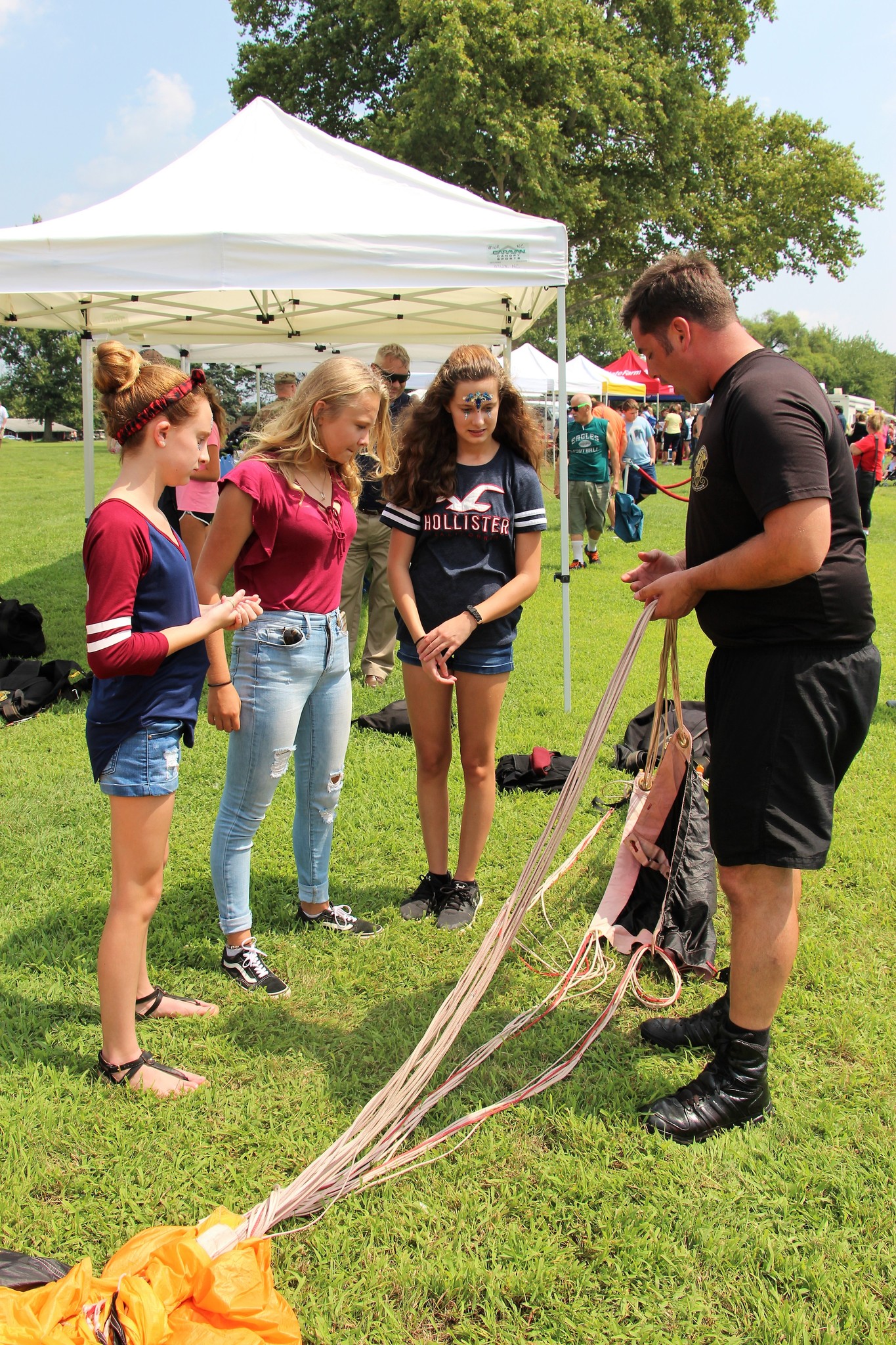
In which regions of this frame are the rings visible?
[228,599,236,608]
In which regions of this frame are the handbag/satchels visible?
[614,492,644,543]
[847,433,857,444]
[890,445,896,455]
[885,436,892,446]
[855,463,875,507]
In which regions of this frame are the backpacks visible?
[642,412,656,429]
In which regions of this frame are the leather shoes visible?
[363,675,384,688]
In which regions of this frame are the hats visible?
[274,372,298,386]
[867,409,874,415]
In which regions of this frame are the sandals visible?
[135,985,219,1023]
[98,1049,212,1100]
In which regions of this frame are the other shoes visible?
[606,526,615,532]
[682,457,689,460]
[638,1022,773,1146]
[662,462,669,466]
[671,463,675,466]
[863,530,869,537]
[640,979,730,1051]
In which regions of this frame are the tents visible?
[496,342,705,472]
[0,93,580,714]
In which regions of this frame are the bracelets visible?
[415,636,424,646]
[466,604,484,625]
[207,680,232,687]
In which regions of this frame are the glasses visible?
[375,364,410,383]
[569,403,591,412]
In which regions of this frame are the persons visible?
[158,374,226,577]
[337,345,420,688]
[74,343,227,1100]
[378,345,547,931]
[194,356,387,1004]
[552,394,620,575]
[618,399,659,507]
[834,405,896,537]
[69,431,78,442]
[247,371,299,446]
[616,249,882,1141]
[0,400,9,450]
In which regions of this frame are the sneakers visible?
[400,870,483,934]
[221,937,291,1000]
[296,901,385,939]
[569,545,602,570]
